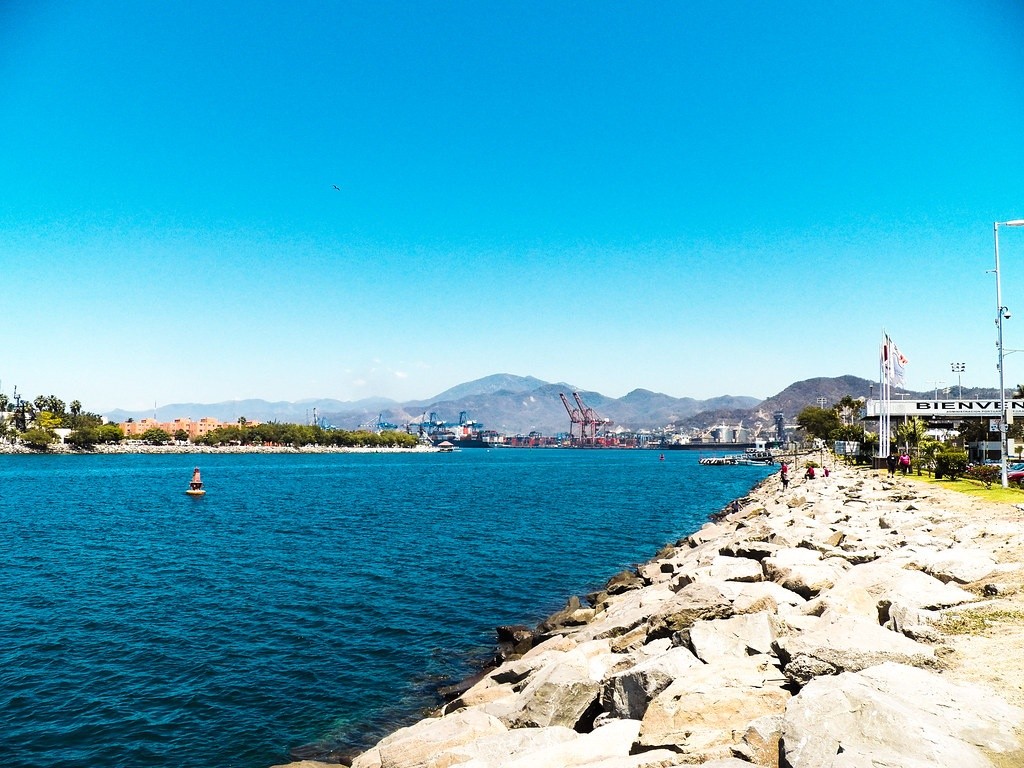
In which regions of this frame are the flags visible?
[882,333,909,389]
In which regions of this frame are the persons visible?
[820,466,829,477]
[886,451,897,478]
[804,465,815,479]
[780,462,788,491]
[898,451,911,476]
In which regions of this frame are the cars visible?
[1008,467,1024,484]
[998,463,1024,480]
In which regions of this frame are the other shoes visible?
[802,477,804,479]
[892,476,894,478]
[804,481,806,483]
[786,486,787,489]
[887,476,890,478]
[903,474,906,478]
[782,490,785,492]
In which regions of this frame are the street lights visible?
[994,220,1024,489]
